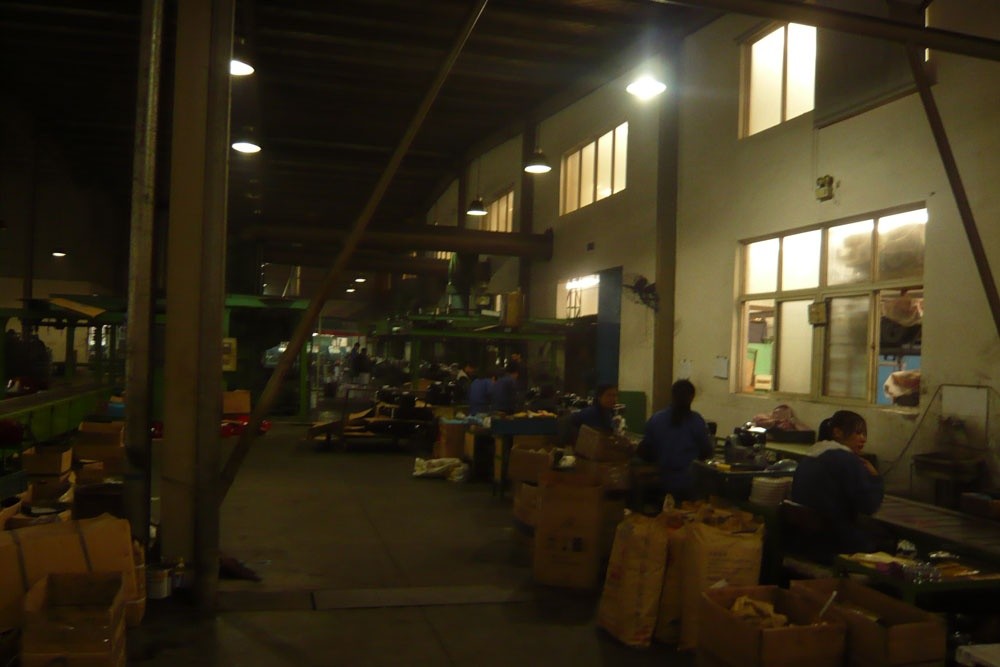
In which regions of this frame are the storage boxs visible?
[510,423,626,594]
[696,576,946,667]
[432,410,465,460]
[0,398,184,667]
[756,424,816,445]
[218,389,251,415]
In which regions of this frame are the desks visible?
[855,489,1000,568]
[688,453,795,501]
[490,415,564,503]
[463,427,491,455]
[765,440,877,474]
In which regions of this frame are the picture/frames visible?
[221,337,238,371]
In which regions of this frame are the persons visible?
[453,349,528,415]
[635,380,714,513]
[572,384,617,429]
[791,410,884,566]
[349,343,370,384]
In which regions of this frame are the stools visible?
[0,419,24,478]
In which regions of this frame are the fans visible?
[619,279,656,309]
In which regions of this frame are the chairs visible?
[617,391,650,436]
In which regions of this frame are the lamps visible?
[344,272,367,293]
[524,123,552,174]
[229,47,258,76]
[815,174,834,201]
[465,158,489,216]
[52,248,67,257]
[231,123,262,153]
[624,54,667,99]
